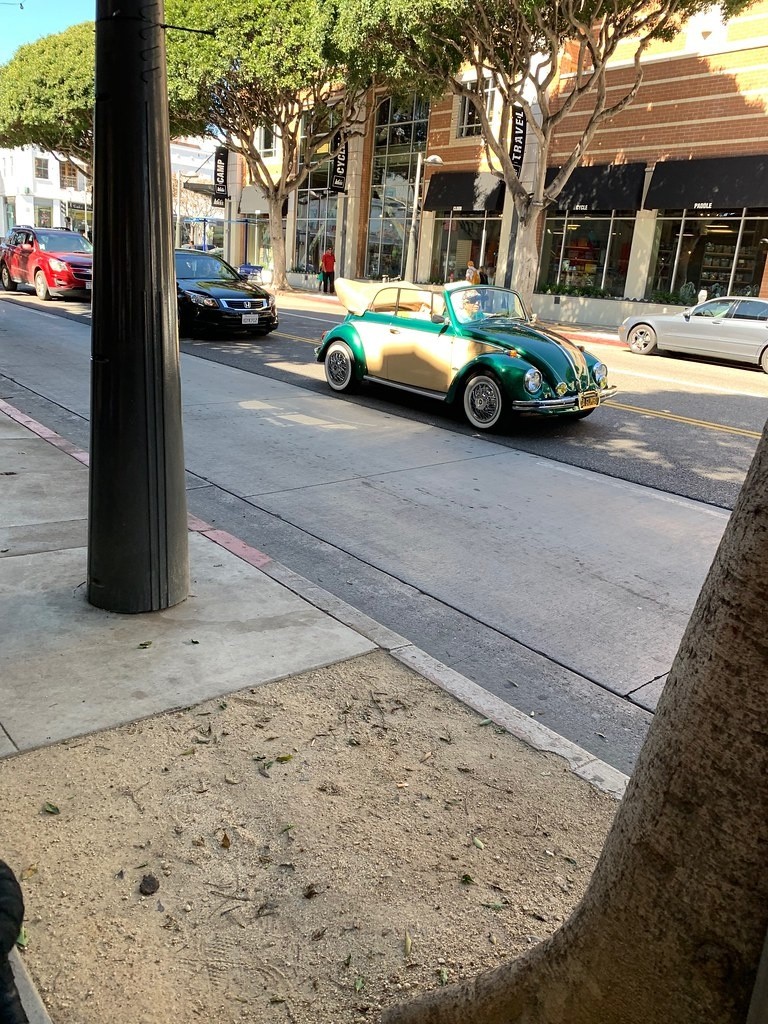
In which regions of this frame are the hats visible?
[467,261,474,266]
[463,290,481,299]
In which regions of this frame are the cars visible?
[173,247,280,338]
[619,295,767,373]
[314,274,619,432]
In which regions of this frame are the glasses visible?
[466,297,481,303]
[328,249,332,250]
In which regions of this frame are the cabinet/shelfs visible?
[546,216,605,291]
[687,228,757,297]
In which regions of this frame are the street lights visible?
[174,169,199,247]
[404,150,442,283]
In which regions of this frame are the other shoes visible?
[324,292,329,294]
[330,293,335,294]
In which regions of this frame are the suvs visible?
[0,224,94,301]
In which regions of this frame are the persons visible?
[317,263,330,291]
[465,261,481,284]
[0,857,32,1022]
[322,247,336,293]
[478,266,487,308]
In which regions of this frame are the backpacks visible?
[471,269,480,284]
